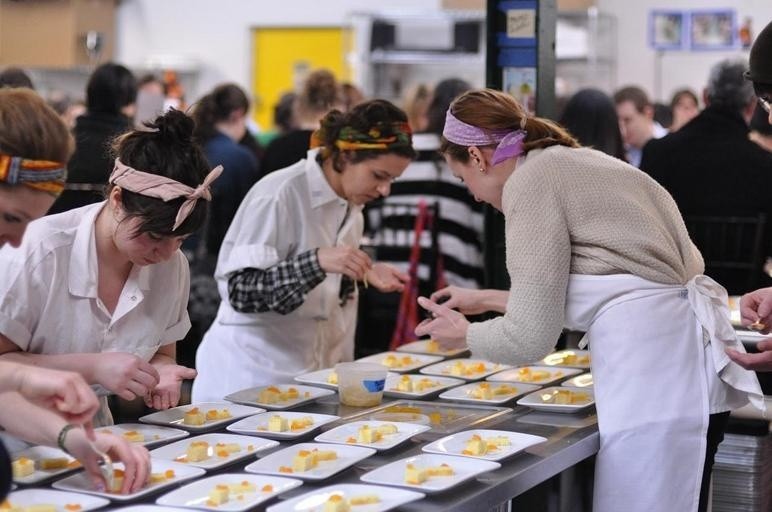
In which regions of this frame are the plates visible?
[291,367,401,392]
[354,351,445,373]
[516,385,596,413]
[220,383,335,411]
[730,326,770,348]
[716,289,758,325]
[360,453,502,495]
[245,443,378,483]
[418,357,518,381]
[538,348,592,370]
[421,428,548,461]
[2,488,111,510]
[148,432,280,469]
[438,381,542,402]
[137,401,266,433]
[396,340,469,356]
[155,473,304,510]
[51,456,206,502]
[263,481,427,511]
[92,421,191,449]
[562,372,594,391]
[8,445,84,485]
[381,375,468,399]
[226,412,341,441]
[314,420,431,450]
[712,434,772,512]
[485,366,585,386]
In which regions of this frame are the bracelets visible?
[52,423,85,452]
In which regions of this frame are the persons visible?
[188,98,419,403]
[414,86,766,512]
[1,67,152,501]
[1,64,772,393]
[724,21,772,373]
[0,102,199,455]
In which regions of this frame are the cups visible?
[332,361,387,407]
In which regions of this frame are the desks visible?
[0,342,598,510]
